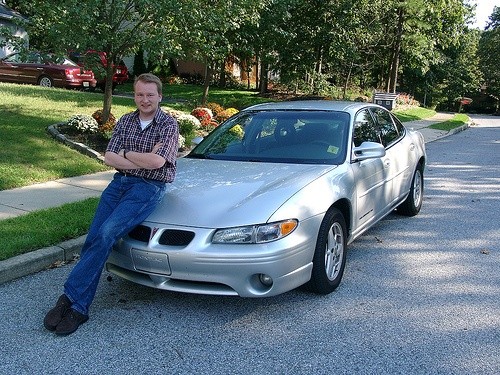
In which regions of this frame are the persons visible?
[43,73,179,336]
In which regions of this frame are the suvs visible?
[66,46,129,93]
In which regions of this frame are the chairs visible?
[301,123,329,144]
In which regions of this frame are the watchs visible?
[123,148,130,158]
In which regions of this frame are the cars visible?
[102,100,428,299]
[0,51,97,89]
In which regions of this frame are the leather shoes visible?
[44,294,72,331]
[55,308,89,335]
